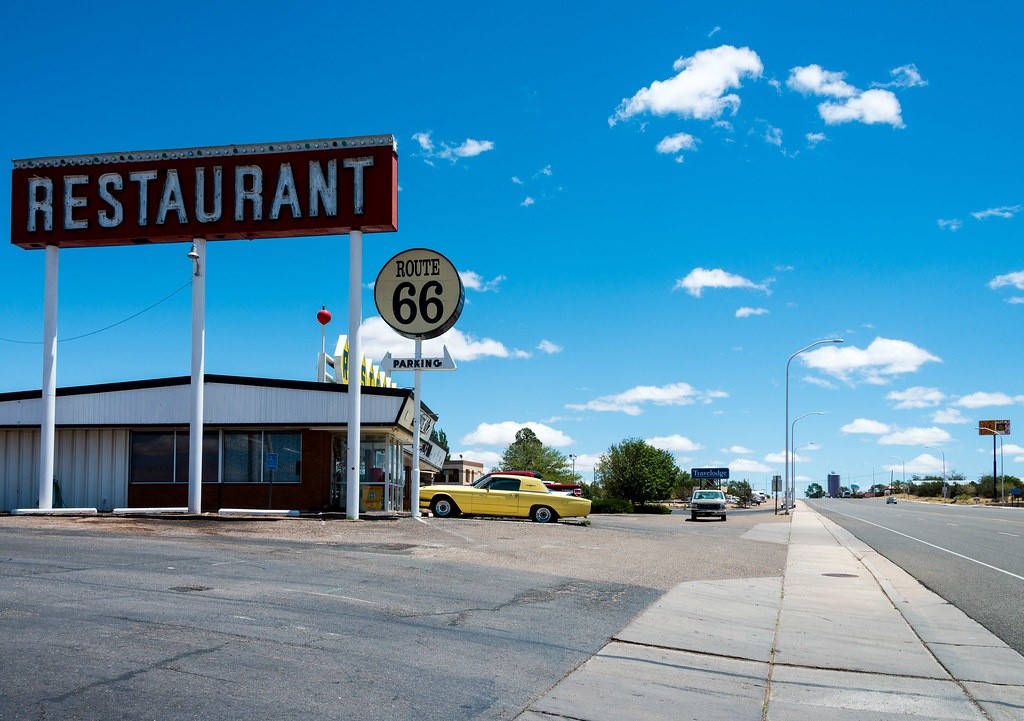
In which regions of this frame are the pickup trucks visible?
[466,471,582,497]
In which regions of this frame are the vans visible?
[842,491,851,497]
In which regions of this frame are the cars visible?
[886,497,897,504]
[727,497,741,504]
[419,474,592,522]
[825,493,830,498]
[687,489,727,521]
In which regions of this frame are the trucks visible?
[752,491,767,503]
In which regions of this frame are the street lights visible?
[186,245,204,515]
[791,412,824,508]
[569,454,578,482]
[792,442,814,502]
[862,463,874,494]
[890,456,905,499]
[974,427,1004,505]
[923,446,945,502]
[784,339,845,514]
